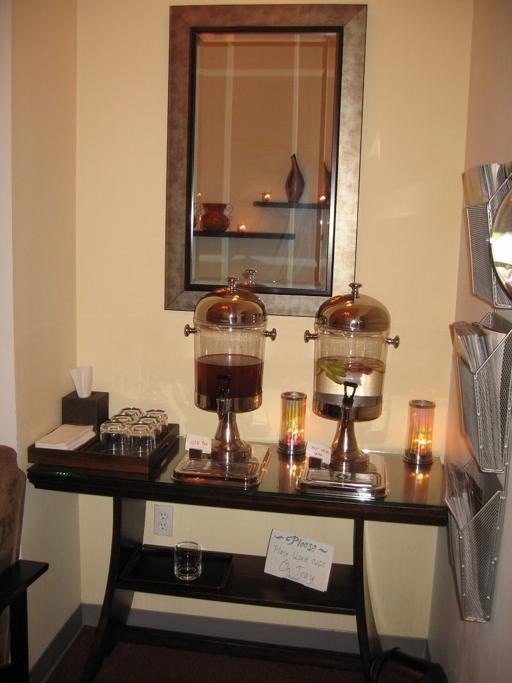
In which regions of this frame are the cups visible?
[100,407,169,458]
[173,541,203,581]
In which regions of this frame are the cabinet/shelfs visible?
[194,200,330,238]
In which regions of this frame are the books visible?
[439,320,506,620]
[36,422,96,450]
[463,162,512,288]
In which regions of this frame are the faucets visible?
[216,372,232,421]
[342,380,356,431]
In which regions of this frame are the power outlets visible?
[154,504,173,537]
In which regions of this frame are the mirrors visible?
[164,5,367,318]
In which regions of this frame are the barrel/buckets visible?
[305,284,399,423]
[184,276,275,410]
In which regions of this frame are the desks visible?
[0,560,50,683]
[27,448,449,683]
